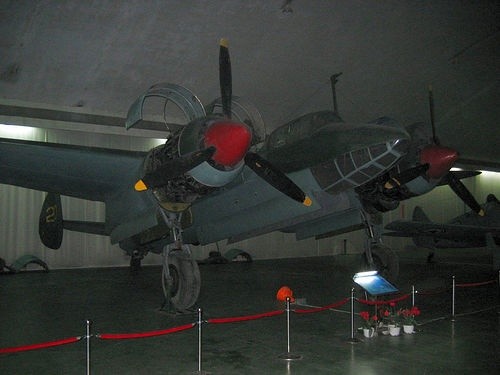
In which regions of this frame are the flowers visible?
[360,303,421,336]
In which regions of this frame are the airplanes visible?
[0,36,500,315]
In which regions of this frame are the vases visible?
[382,325,395,335]
[390,327,400,336]
[363,328,374,338]
[403,325,414,334]
